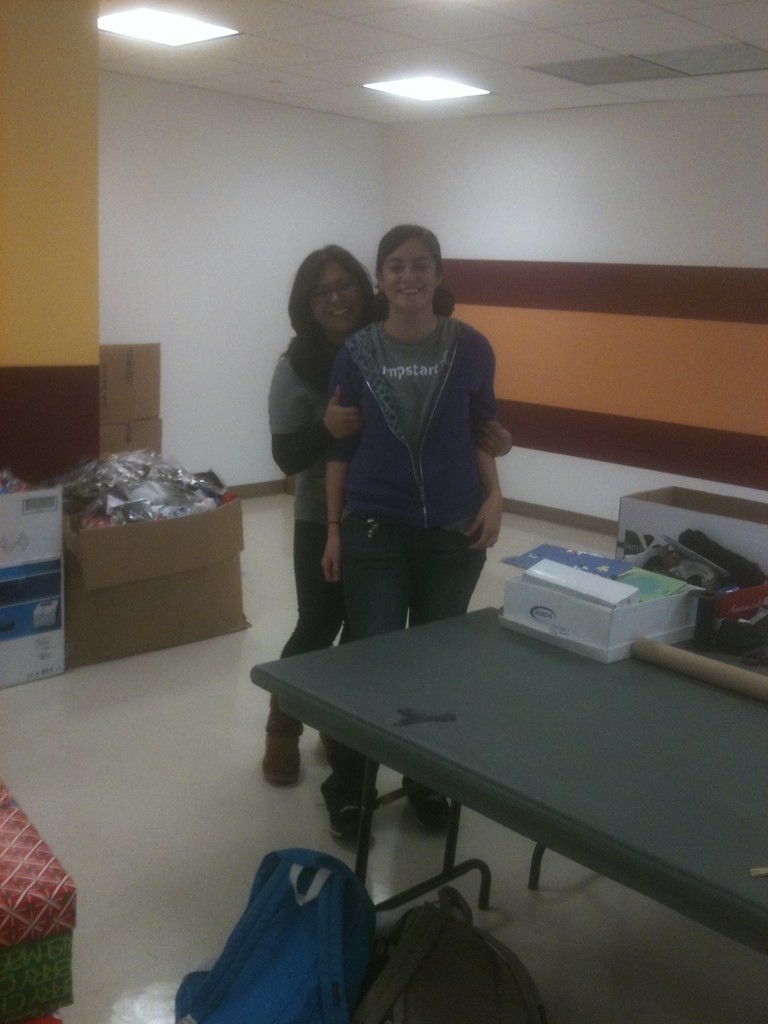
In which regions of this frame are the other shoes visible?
[328,796,370,844]
[406,786,450,833]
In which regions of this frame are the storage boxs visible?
[615,485,768,579]
[0,778,77,1024]
[0,343,252,686]
[499,555,704,665]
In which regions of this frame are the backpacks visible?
[351,884,550,1024]
[174,847,378,1024]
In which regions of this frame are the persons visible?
[261,224,513,849]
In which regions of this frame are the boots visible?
[260,643,306,785]
[313,730,365,783]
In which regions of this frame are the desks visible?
[248,604,768,957]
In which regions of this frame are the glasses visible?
[305,274,361,304]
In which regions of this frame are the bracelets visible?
[327,522,341,526]
